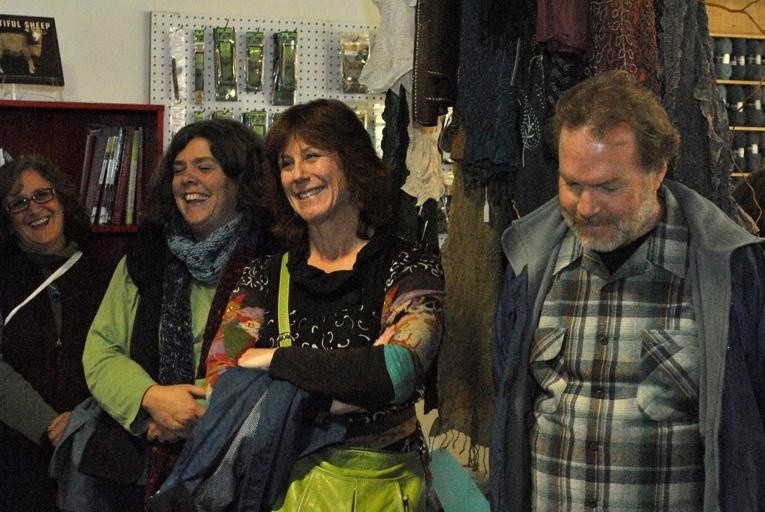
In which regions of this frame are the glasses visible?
[7,186,55,219]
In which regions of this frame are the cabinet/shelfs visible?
[0,99,164,277]
[707,31,765,177]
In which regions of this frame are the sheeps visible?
[1,24,49,75]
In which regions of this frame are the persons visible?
[0,151,112,511]
[82,117,265,511]
[204,99,447,510]
[485,65,763,510]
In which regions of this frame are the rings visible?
[187,422,194,430]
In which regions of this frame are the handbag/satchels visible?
[268,443,427,512]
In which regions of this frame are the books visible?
[77,119,144,228]
[0,14,64,92]
